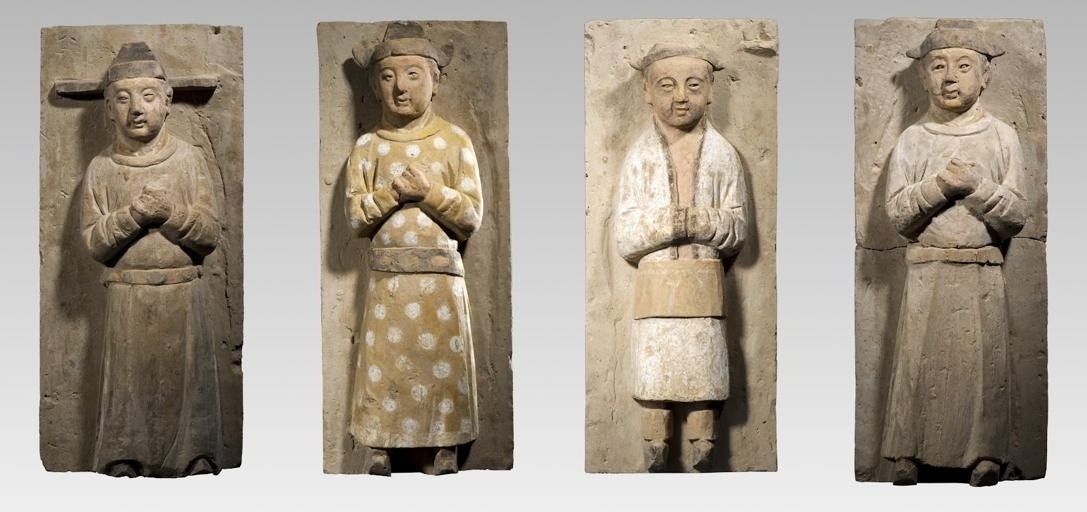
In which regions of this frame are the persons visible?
[334,22,488,476]
[872,21,1031,488]
[608,36,754,472]
[51,40,230,476]
